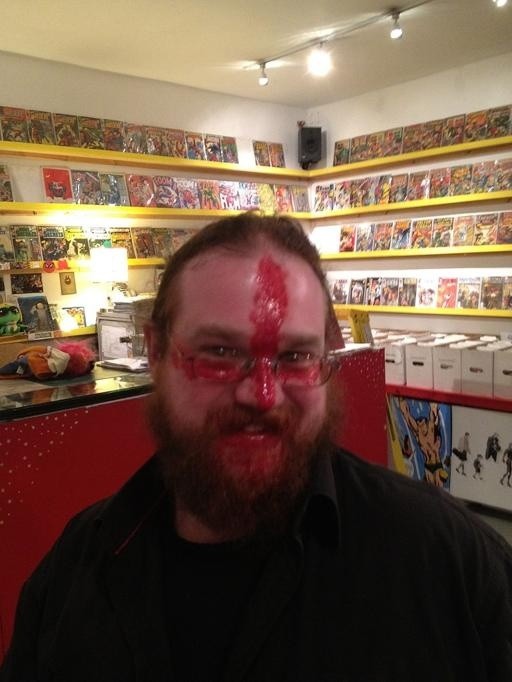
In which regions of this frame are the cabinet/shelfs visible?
[0,140,312,373]
[313,132,512,319]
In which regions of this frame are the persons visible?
[0,215,512,682]
[399,396,444,488]
[456,432,512,487]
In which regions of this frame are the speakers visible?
[298,127,321,164]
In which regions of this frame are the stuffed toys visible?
[0,343,96,382]
[0,303,28,336]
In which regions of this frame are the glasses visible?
[168,335,334,388]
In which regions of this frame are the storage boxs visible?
[335,319,511,404]
